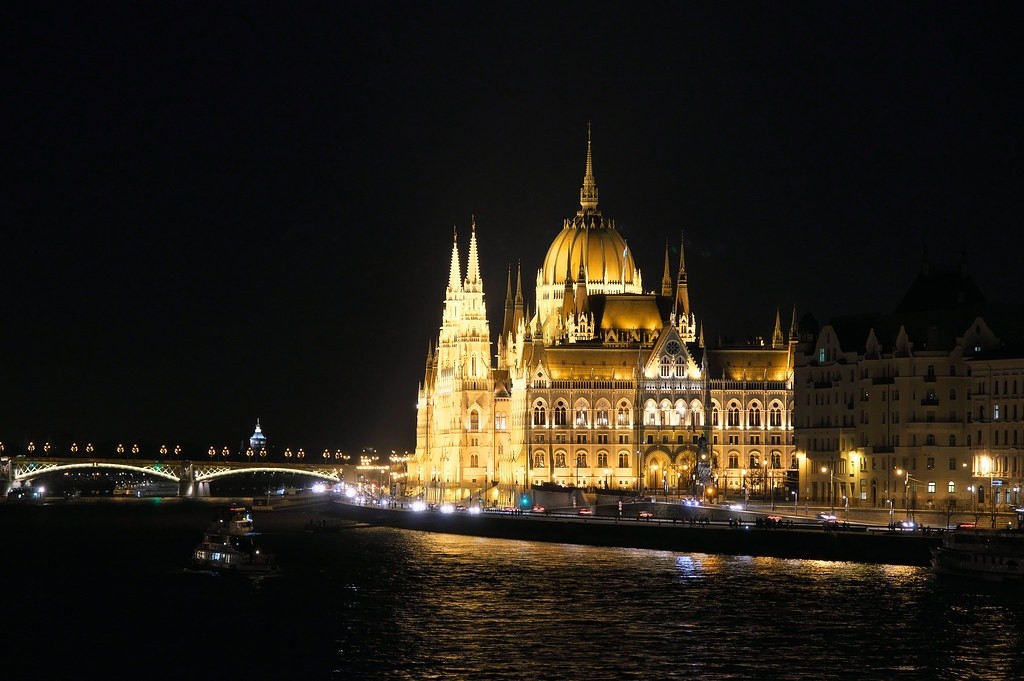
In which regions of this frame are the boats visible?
[184,504,284,591]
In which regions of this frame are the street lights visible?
[160,445,168,459]
[27,441,35,458]
[886,500,893,525]
[132,444,138,459]
[207,446,216,461]
[117,443,124,459]
[259,448,267,461]
[284,447,293,463]
[843,495,849,520]
[323,449,331,464]
[334,449,342,465]
[71,443,78,458]
[790,490,798,515]
[86,442,94,459]
[174,446,181,460]
[222,447,230,461]
[43,442,50,457]
[246,447,254,463]
[297,448,305,462]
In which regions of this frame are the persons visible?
[728,516,793,531]
[887,522,932,534]
[618,511,710,528]
[823,520,851,535]
